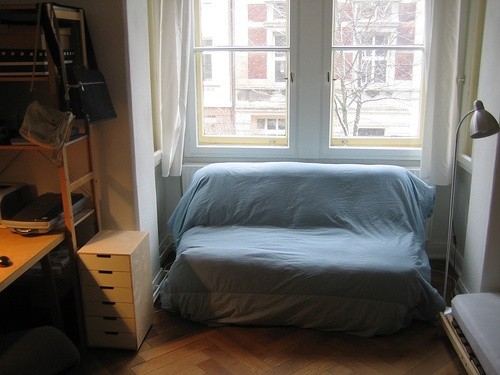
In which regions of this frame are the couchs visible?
[159,162,447,338]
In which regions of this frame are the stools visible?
[438,293,500,375]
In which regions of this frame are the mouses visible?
[0,256,12,268]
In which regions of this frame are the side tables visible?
[76,230,154,350]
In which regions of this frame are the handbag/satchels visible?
[67,69,117,123]
[19,101,76,150]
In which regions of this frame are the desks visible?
[0,207,94,334]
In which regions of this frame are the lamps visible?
[429,100,500,328]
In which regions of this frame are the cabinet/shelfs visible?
[0,1,102,357]
